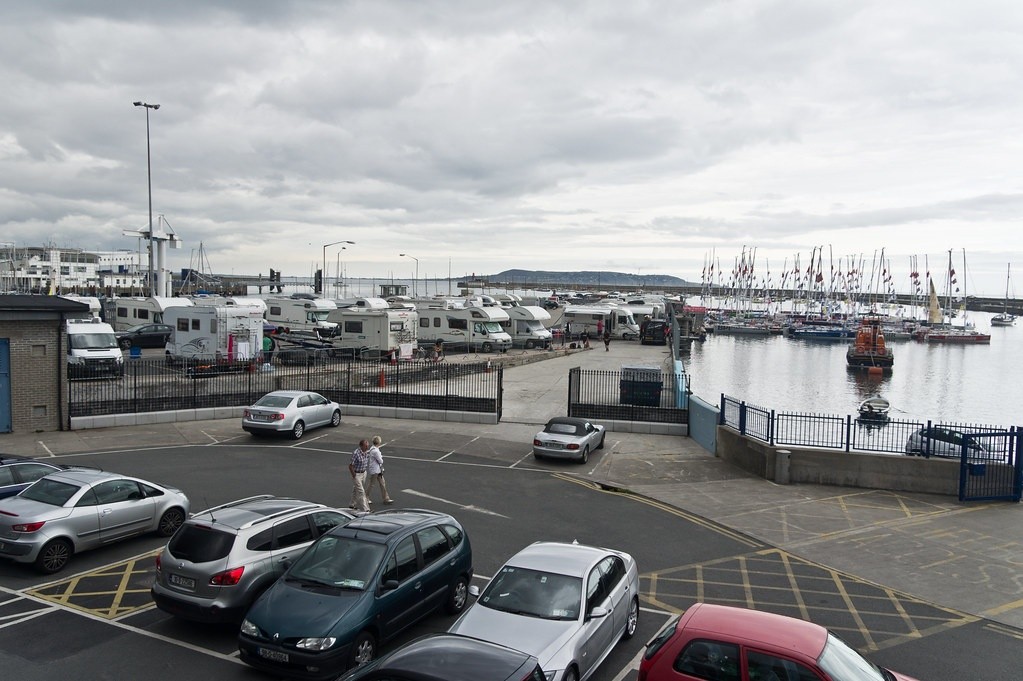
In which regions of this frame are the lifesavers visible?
[857,346,865,353]
[877,347,886,355]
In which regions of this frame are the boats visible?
[857,393,891,424]
[844,311,895,375]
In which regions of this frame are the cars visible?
[0,453,105,504]
[0,466,192,575]
[904,422,1016,465]
[241,389,343,440]
[444,540,641,681]
[115,322,173,350]
[334,630,547,681]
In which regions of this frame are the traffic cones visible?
[376,368,388,388]
[485,356,494,373]
[388,347,399,366]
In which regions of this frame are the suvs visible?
[237,507,475,680]
[150,494,377,630]
[639,318,670,346]
[637,600,918,681]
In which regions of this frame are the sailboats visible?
[989,261,1021,327]
[701,244,992,345]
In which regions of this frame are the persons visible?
[348,440,371,513]
[604,331,610,351]
[367,435,394,503]
[263,333,275,362]
[597,319,604,341]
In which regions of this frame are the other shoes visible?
[383,499,394,505]
[368,500,373,504]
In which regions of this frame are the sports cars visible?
[532,416,606,464]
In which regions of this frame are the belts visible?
[355,469,365,473]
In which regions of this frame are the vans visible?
[65,288,688,381]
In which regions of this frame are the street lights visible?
[323,241,356,300]
[400,253,419,299]
[132,101,161,297]
[336,247,347,300]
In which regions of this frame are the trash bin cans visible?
[775,450,793,484]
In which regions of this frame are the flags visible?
[699,263,959,312]
